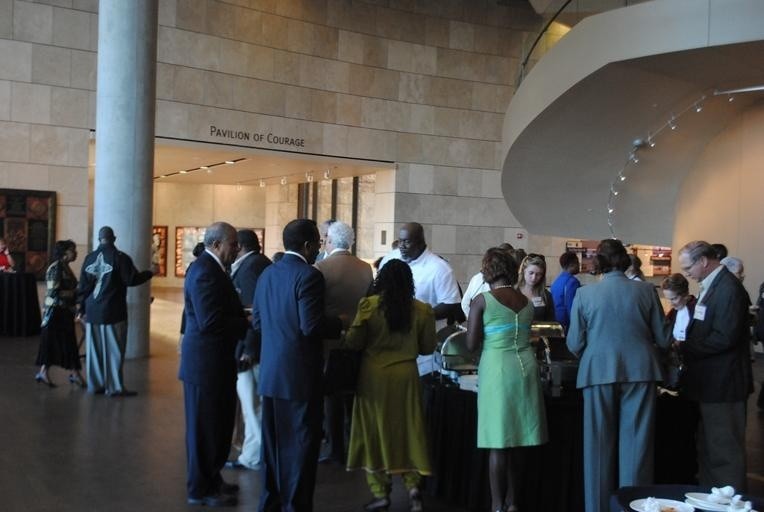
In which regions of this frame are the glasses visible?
[525,253,545,264]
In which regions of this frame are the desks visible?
[0,270,43,338]
[418,365,587,512]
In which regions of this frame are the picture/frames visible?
[0,186,58,283]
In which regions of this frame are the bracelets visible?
[5,253,10,257]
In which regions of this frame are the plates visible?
[628,497,695,512]
[684,491,747,511]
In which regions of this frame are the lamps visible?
[712,82,763,104]
[231,163,337,193]
[603,92,709,244]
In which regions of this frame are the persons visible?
[0,238,16,270]
[34,239,87,389]
[177,218,763,511]
[75,226,160,398]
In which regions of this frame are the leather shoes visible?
[225,460,249,472]
[409,488,423,512]
[186,493,238,506]
[365,496,389,509]
[108,388,138,396]
[218,482,240,493]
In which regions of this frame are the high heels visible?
[69,374,87,388]
[36,373,58,388]
